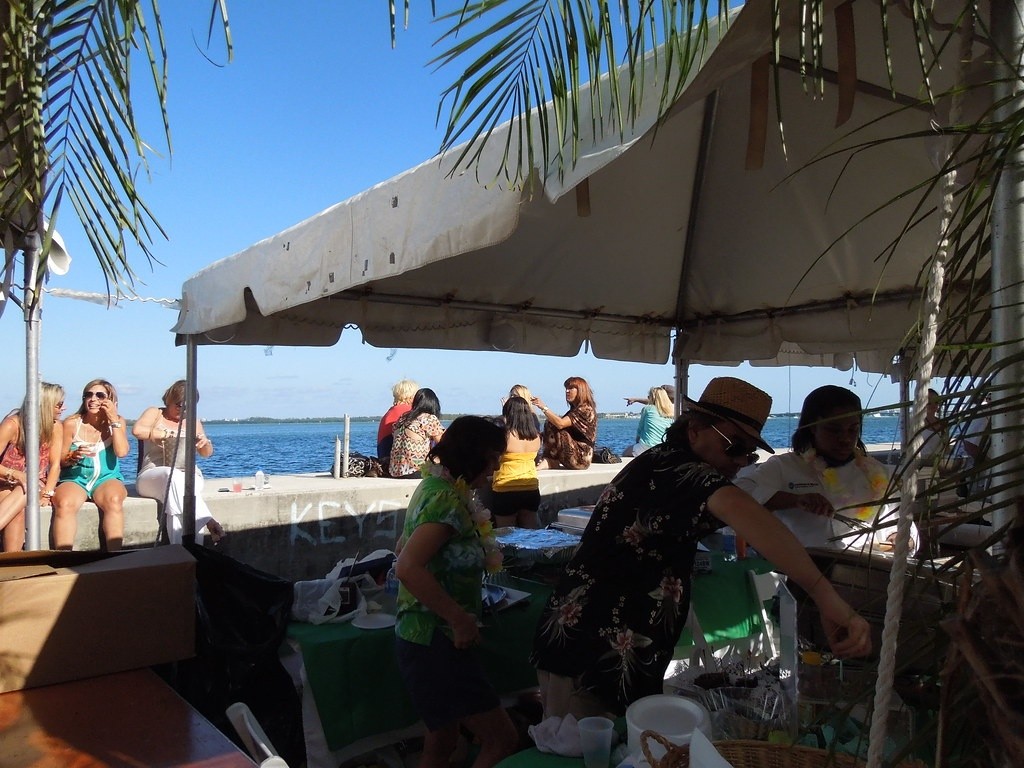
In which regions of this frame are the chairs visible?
[226,701,289,768]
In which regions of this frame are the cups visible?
[578,717,614,767]
[85,443,98,456]
[233,476,243,494]
[264,474,271,489]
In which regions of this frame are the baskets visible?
[640,730,868,768]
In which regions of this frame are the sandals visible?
[207,523,225,544]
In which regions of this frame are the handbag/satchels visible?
[592,446,622,464]
[184,542,294,651]
[291,578,368,625]
[365,456,390,478]
[165,438,198,469]
[330,451,369,478]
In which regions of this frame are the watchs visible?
[208,439,211,444]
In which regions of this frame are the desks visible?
[0,553,907,768]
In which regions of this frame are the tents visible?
[170,0,1024,550]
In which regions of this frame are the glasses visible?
[175,403,186,411]
[56,401,63,409]
[487,454,502,464]
[84,392,109,400]
[710,424,759,466]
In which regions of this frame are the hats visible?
[682,378,775,454]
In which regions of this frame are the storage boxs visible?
[0,542,197,693]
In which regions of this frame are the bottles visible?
[384,561,399,614]
[255,467,264,491]
[797,651,826,732]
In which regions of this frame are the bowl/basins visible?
[695,686,783,739]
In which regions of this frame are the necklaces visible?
[421,462,503,574]
[89,419,103,438]
[797,442,890,523]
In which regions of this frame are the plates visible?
[482,583,507,608]
[353,613,396,629]
[626,693,712,762]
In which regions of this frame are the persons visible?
[491,397,542,529]
[919,389,992,509]
[0,381,226,552]
[396,416,519,768]
[535,377,871,720]
[733,385,920,568]
[378,377,676,478]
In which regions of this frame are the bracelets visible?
[542,405,549,414]
[163,428,170,440]
[112,422,122,428]
[42,490,54,497]
[5,469,15,479]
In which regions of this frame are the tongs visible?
[804,504,872,531]
